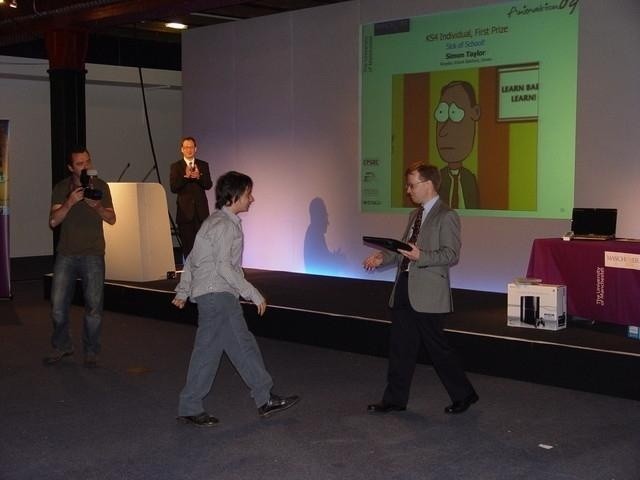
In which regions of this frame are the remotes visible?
[563,231,574,240]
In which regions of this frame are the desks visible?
[525,237,640,328]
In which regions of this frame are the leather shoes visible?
[444,396,479,414]
[368,400,406,415]
[257,395,300,419]
[176,412,221,428]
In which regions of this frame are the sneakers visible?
[43,345,74,364]
[83,355,97,368]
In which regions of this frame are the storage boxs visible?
[506,280,568,332]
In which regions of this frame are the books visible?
[362,236,411,254]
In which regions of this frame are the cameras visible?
[142,165,156,181]
[81,169,106,200]
[117,162,130,181]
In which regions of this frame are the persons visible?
[44,145,117,369]
[168,171,302,430]
[168,136,213,265]
[358,161,480,415]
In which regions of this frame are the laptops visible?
[561,206,618,241]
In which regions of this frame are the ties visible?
[400,206,424,271]
